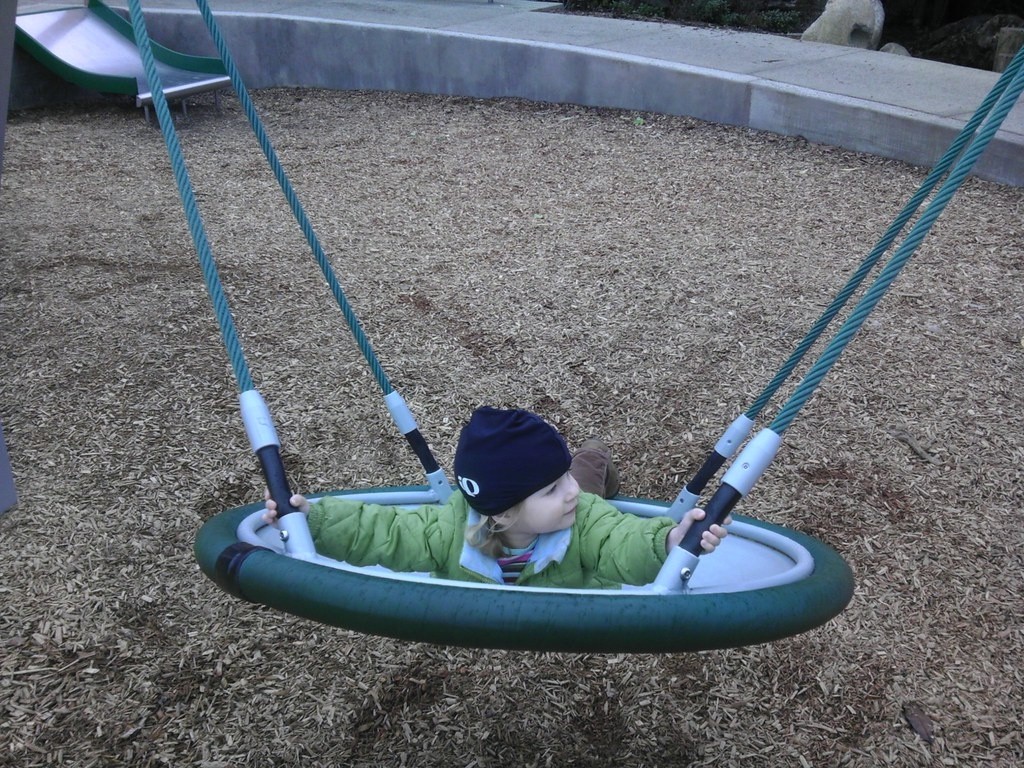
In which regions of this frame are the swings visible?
[122,1,1023,654]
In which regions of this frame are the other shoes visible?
[581,438,620,498]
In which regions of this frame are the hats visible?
[454,406,572,515]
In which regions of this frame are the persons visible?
[262,405,732,590]
